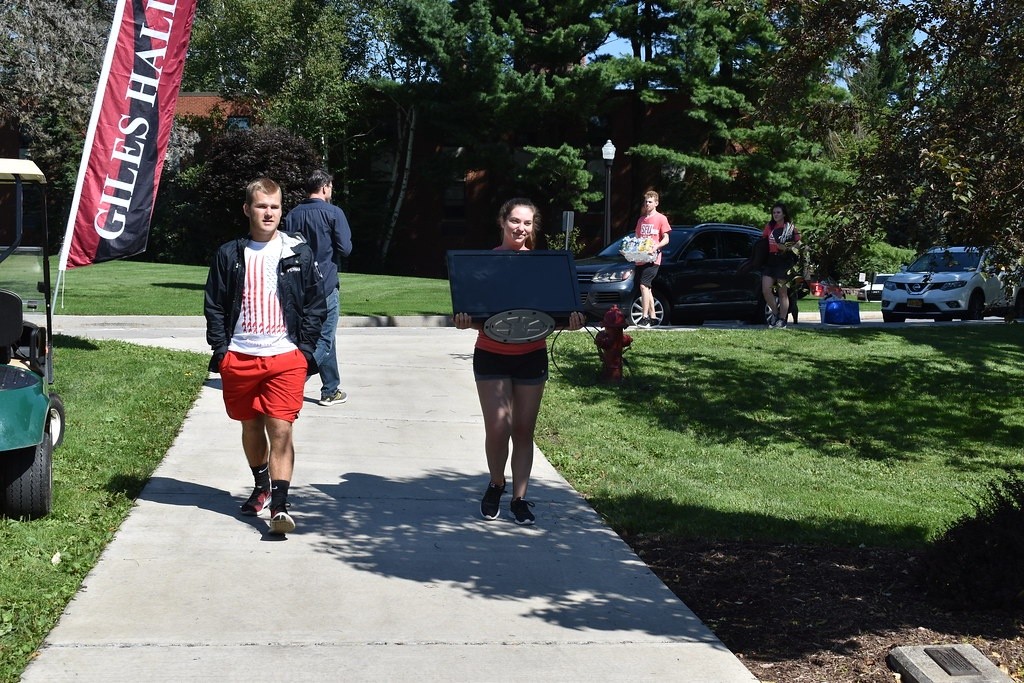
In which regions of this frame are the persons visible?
[454,199,585,526]
[636,191,672,328]
[204,177,325,533]
[762,202,802,329]
[284,169,352,408]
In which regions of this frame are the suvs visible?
[547,222,790,329]
[880,246,1024,324]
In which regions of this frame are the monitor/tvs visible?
[445,250,584,344]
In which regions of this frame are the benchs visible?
[0,290,30,370]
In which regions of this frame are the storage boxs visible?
[818,301,860,324]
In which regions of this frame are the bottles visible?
[622,236,658,262]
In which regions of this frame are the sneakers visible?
[267,502,296,533]
[774,318,787,329]
[319,388,348,405]
[637,318,651,328]
[769,316,779,328]
[480,477,507,519]
[241,482,273,516]
[649,318,660,328]
[508,496,536,524]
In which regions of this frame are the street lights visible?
[601,140,616,248]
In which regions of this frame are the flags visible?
[60,0,198,273]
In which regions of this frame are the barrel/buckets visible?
[819,302,826,324]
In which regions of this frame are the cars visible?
[857,273,895,301]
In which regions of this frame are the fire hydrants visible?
[595,303,634,384]
